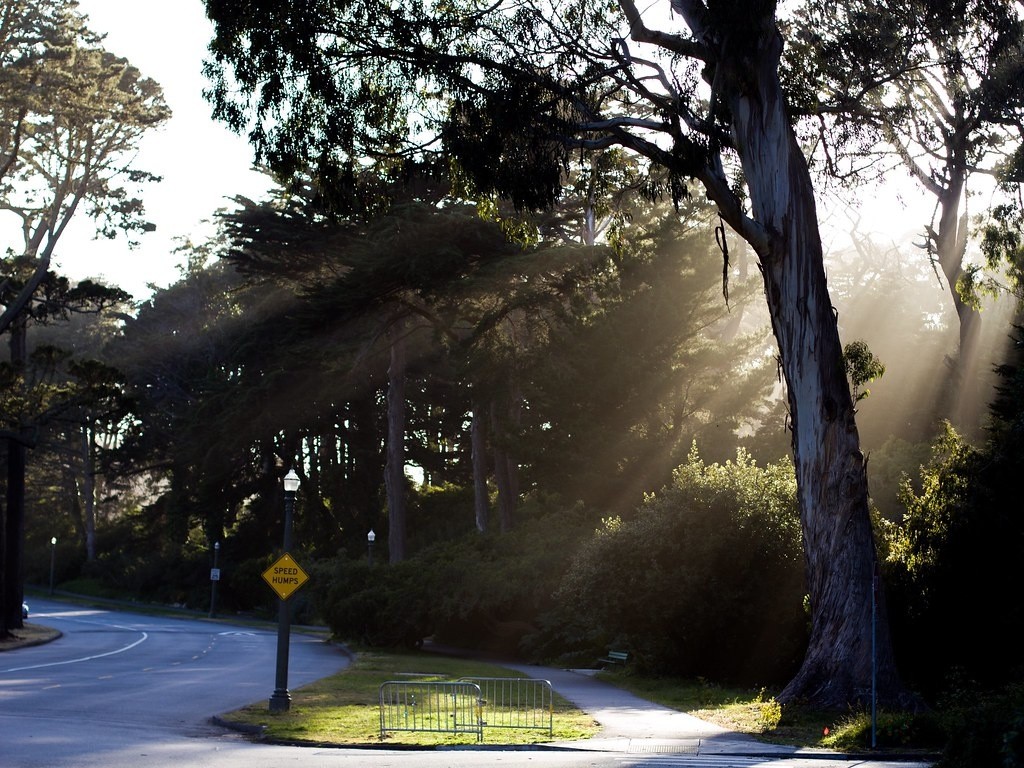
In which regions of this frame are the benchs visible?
[598,650,628,670]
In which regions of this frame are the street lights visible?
[46,536,57,596]
[208,540,220,619]
[269,463,302,714]
[366,529,375,567]
